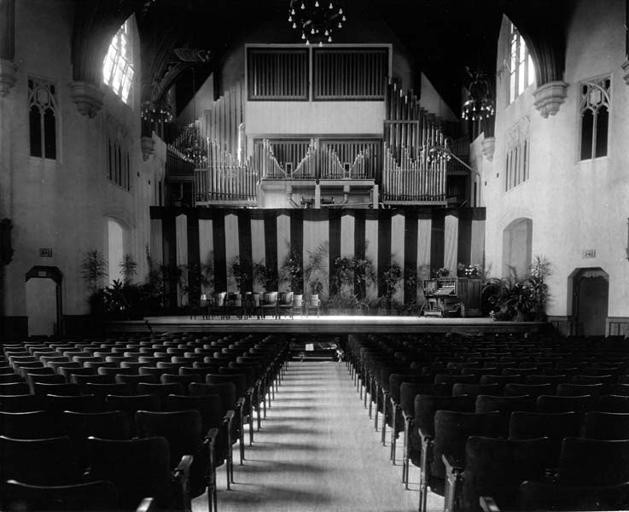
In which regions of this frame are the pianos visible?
[423,278,468,317]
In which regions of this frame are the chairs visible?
[347,327,629,512]
[0,331,290,512]
[189,291,321,320]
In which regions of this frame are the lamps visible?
[140,99,175,126]
[287,0,347,48]
[183,69,208,164]
[460,70,496,121]
